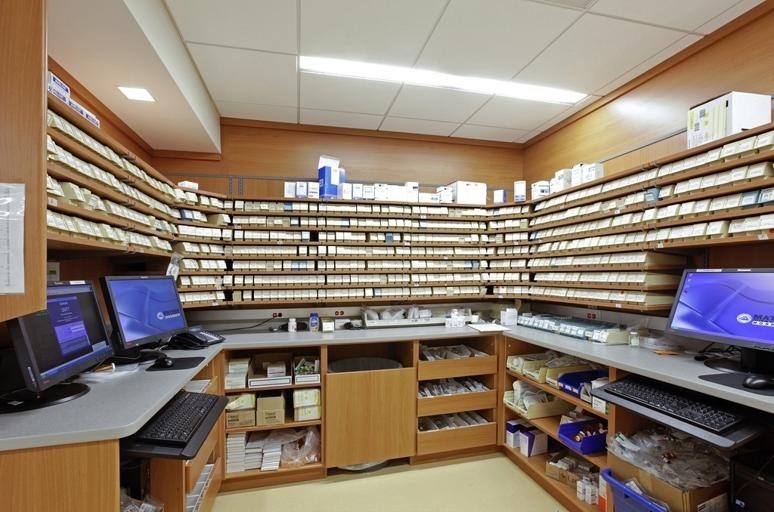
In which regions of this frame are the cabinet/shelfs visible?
[415,333,502,466]
[44,87,774,314]
[220,347,324,493]
[500,329,609,512]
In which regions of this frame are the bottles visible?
[627,331,640,347]
[444,316,451,328]
[287,318,296,331]
[308,312,318,331]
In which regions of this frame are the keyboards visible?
[604,375,745,434]
[138,391,220,445]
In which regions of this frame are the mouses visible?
[155,357,175,367]
[743,376,774,390]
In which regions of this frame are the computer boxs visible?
[730,448,774,512]
[120,457,151,501]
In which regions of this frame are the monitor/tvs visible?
[101,275,188,365]
[0,280,115,413]
[663,268,774,375]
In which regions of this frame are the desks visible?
[0,329,225,511]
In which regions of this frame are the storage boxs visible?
[505,417,548,458]
[598,466,669,511]
[223,353,321,430]
[604,429,730,511]
[545,447,598,487]
